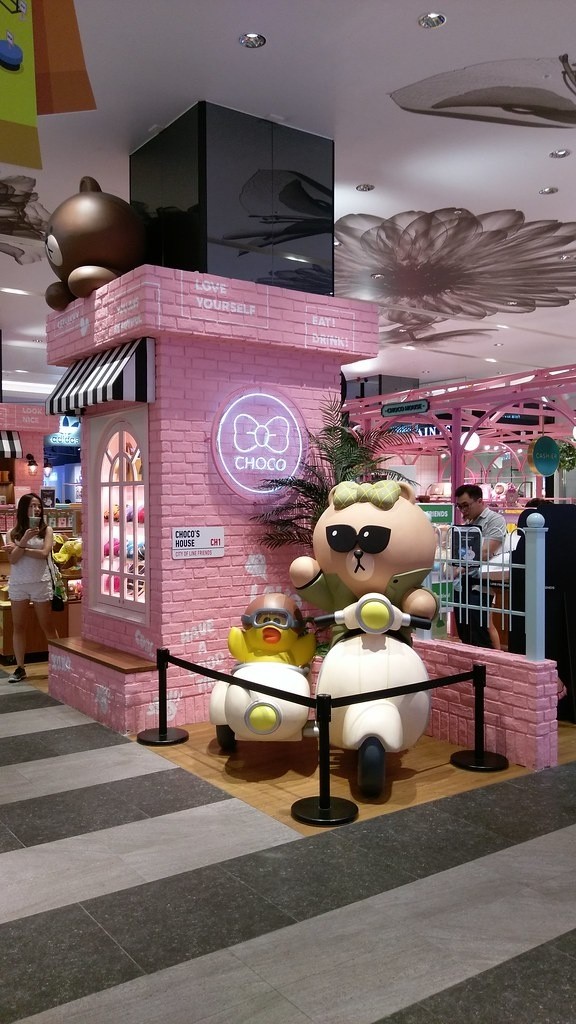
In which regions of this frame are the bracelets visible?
[17,545,26,549]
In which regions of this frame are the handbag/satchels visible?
[50,593,65,612]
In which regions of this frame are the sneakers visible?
[7,666,27,682]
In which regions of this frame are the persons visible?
[1,493,61,694]
[451,483,508,650]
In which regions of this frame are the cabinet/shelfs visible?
[0,508,82,663]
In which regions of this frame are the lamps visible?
[43,458,52,476]
[63,416,81,428]
[26,454,38,476]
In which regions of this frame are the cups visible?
[30,517,41,528]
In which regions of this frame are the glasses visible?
[454,501,476,511]
